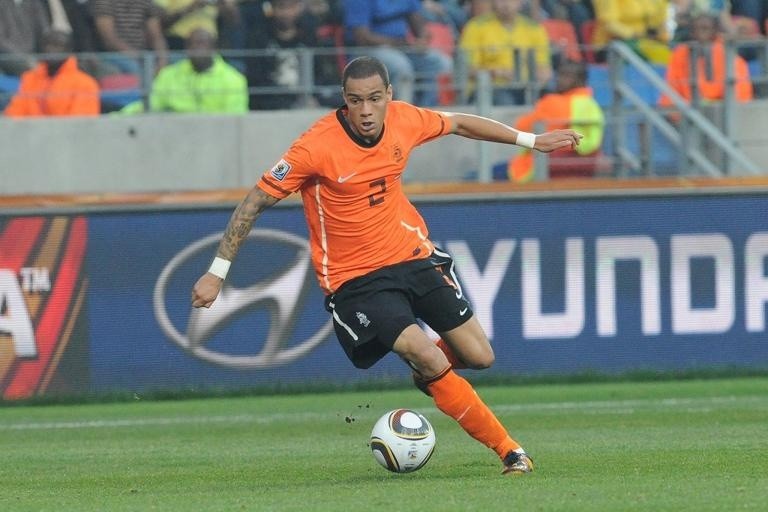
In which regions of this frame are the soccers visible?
[371,409,435,472]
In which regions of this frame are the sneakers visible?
[499,447,534,477]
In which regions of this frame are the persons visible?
[190,56,582,473]
[404,0,768,125]
[1,1,454,126]
[517,60,606,181]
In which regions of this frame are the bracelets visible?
[515,131,537,149]
[208,256,232,281]
